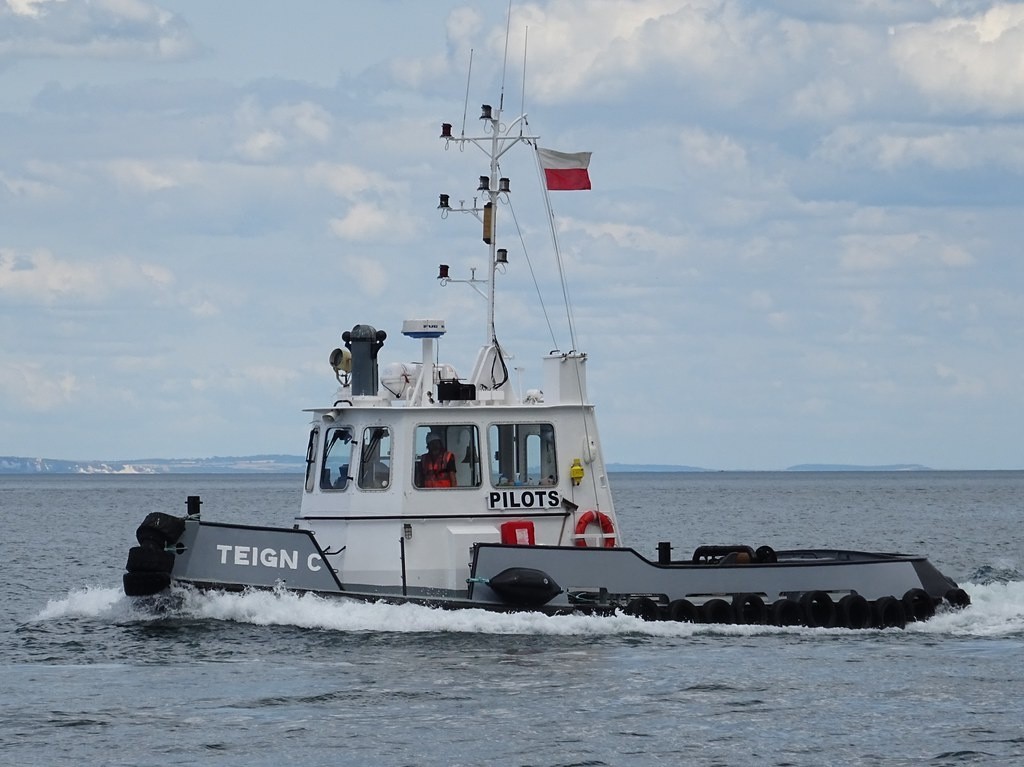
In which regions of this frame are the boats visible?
[122,2,970,633]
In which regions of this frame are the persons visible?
[414,431,459,490]
[537,146,595,192]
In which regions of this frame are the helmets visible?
[425,432,442,443]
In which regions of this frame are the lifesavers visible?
[576,510,615,548]
